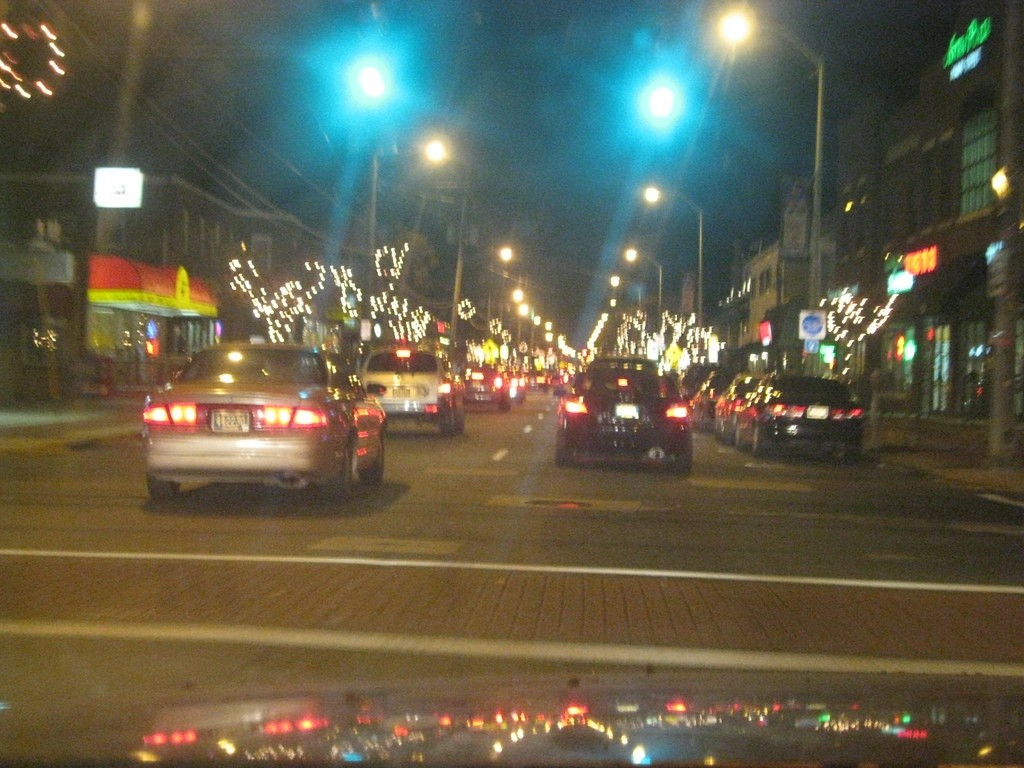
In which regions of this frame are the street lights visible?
[722,5,825,377]
[421,136,471,372]
[644,185,705,365]
[626,249,664,364]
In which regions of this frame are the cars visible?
[682,364,817,451]
[553,355,695,475]
[461,359,574,412]
[740,375,865,461]
[142,340,387,506]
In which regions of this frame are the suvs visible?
[360,344,464,439]
